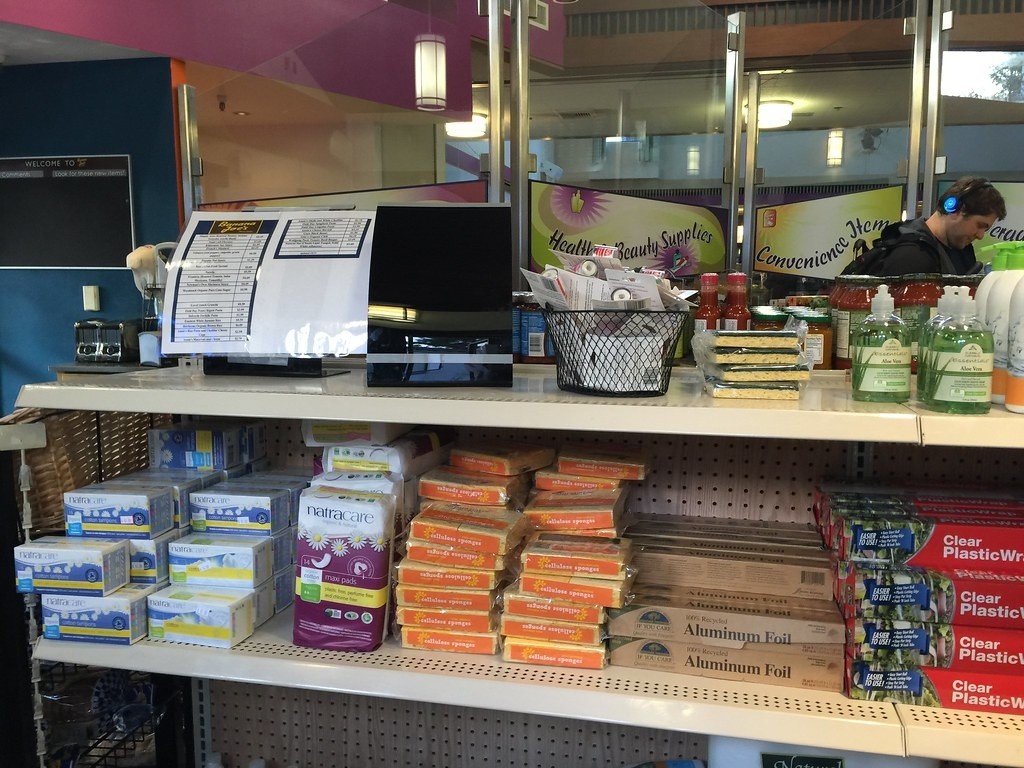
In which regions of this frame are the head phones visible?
[943,178,993,213]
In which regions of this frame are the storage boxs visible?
[395,439,653,671]
[814,476,1024,715]
[610,510,847,693]
[13,423,316,651]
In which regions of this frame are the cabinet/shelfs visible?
[896,374,1024,768]
[14,363,921,768]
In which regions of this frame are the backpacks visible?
[840,234,953,276]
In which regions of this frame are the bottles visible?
[693,272,723,331]
[723,272,752,330]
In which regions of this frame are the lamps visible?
[744,99,794,130]
[827,128,843,167]
[414,0,447,112]
[445,113,488,139]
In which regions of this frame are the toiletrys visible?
[607,480,1024,719]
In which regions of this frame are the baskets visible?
[542,305,689,395]
[0,404,173,534]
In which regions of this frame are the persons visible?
[863,175,1006,277]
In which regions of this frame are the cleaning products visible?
[843,237,1024,416]
